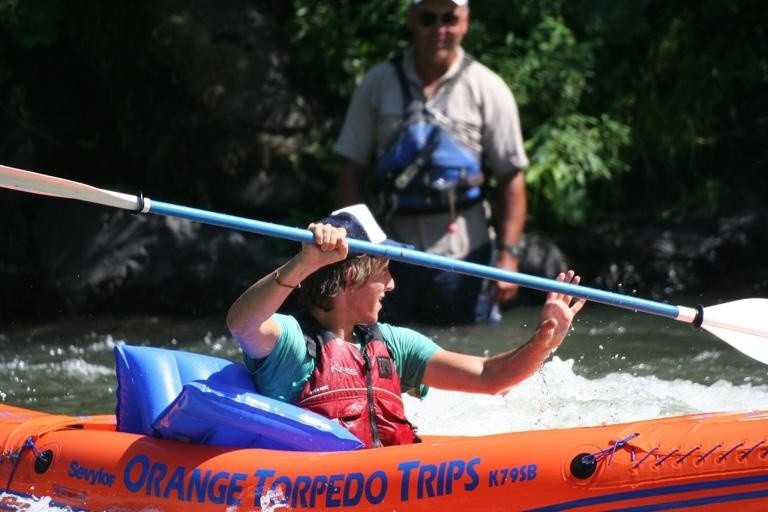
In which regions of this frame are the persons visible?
[225,204,589,449]
[330,1,530,328]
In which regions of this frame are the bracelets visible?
[274,267,303,290]
[499,241,525,262]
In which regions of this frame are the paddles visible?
[0,164,768,365]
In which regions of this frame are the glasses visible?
[418,9,461,27]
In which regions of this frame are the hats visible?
[316,203,416,253]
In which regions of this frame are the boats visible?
[0,378,768,512]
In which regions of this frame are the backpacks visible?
[367,50,487,217]
